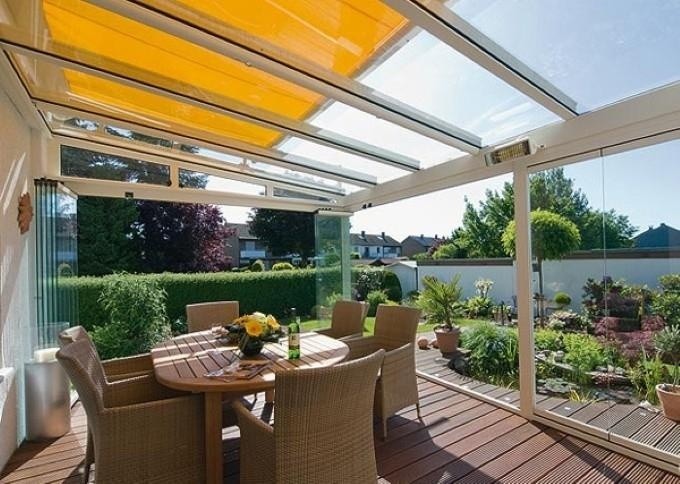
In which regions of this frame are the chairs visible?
[232,349,383,483]
[56,325,204,484]
[186,303,243,332]
[313,297,422,438]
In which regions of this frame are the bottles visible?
[287,307,301,360]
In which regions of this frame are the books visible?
[204,361,270,383]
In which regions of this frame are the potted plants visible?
[651,324,679,422]
[414,273,461,353]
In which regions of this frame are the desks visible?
[149,327,348,482]
